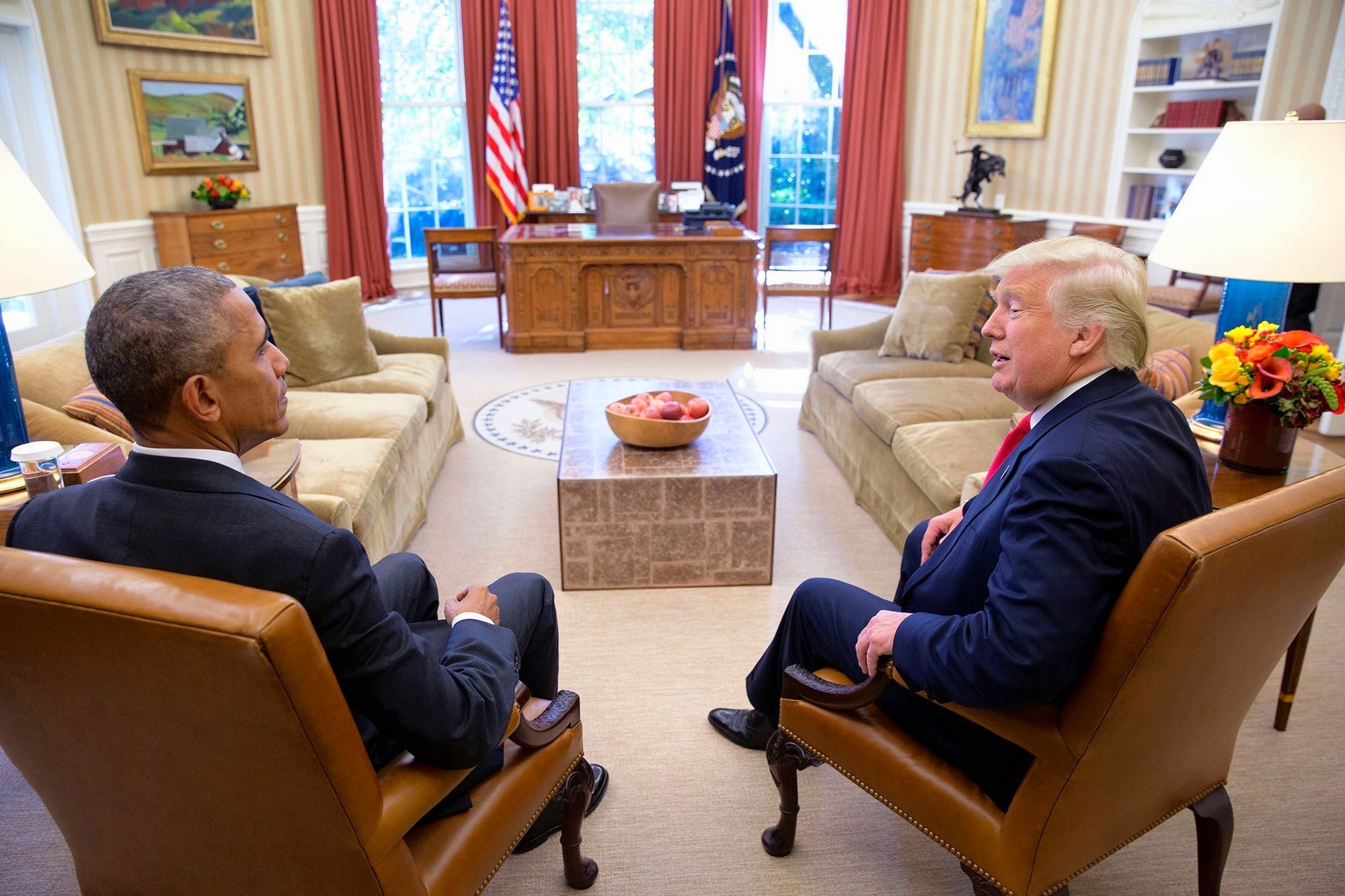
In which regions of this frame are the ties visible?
[979,413,1032,492]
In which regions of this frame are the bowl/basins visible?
[604,390,712,449]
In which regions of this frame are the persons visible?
[708,236,1213,817]
[956,145,992,183]
[6,268,609,854]
[1283,103,1325,330]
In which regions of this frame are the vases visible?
[1218,400,1299,475]
[207,196,237,209]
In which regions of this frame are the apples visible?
[608,391,708,421]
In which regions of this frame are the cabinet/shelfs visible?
[148,205,327,286]
[1103,8,1342,267]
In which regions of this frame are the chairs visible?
[0,546,599,896]
[593,181,661,230]
[762,461,1345,896]
[422,226,506,349]
[1067,221,1128,247]
[1144,270,1225,319]
[756,225,839,332]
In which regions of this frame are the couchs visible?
[798,274,1032,556]
[12,274,466,569]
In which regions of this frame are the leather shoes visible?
[512,764,610,855]
[708,709,778,751]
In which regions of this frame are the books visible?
[1164,100,1226,129]
[1124,185,1163,219]
[1227,47,1265,81]
[1136,58,1180,85]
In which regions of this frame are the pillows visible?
[878,269,990,365]
[61,382,135,444]
[240,271,330,374]
[257,276,384,387]
[925,268,1001,360]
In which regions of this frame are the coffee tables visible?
[555,379,778,591]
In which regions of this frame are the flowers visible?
[1189,321,1345,430]
[190,173,252,204]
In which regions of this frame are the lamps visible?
[1146,117,1345,443]
[0,136,93,474]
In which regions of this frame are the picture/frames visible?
[964,1,1059,137]
[127,68,260,176]
[88,0,273,58]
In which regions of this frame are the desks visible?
[497,223,759,354]
[906,212,1046,283]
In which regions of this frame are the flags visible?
[703,0,745,220]
[485,0,530,224]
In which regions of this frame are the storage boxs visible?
[59,442,125,487]
